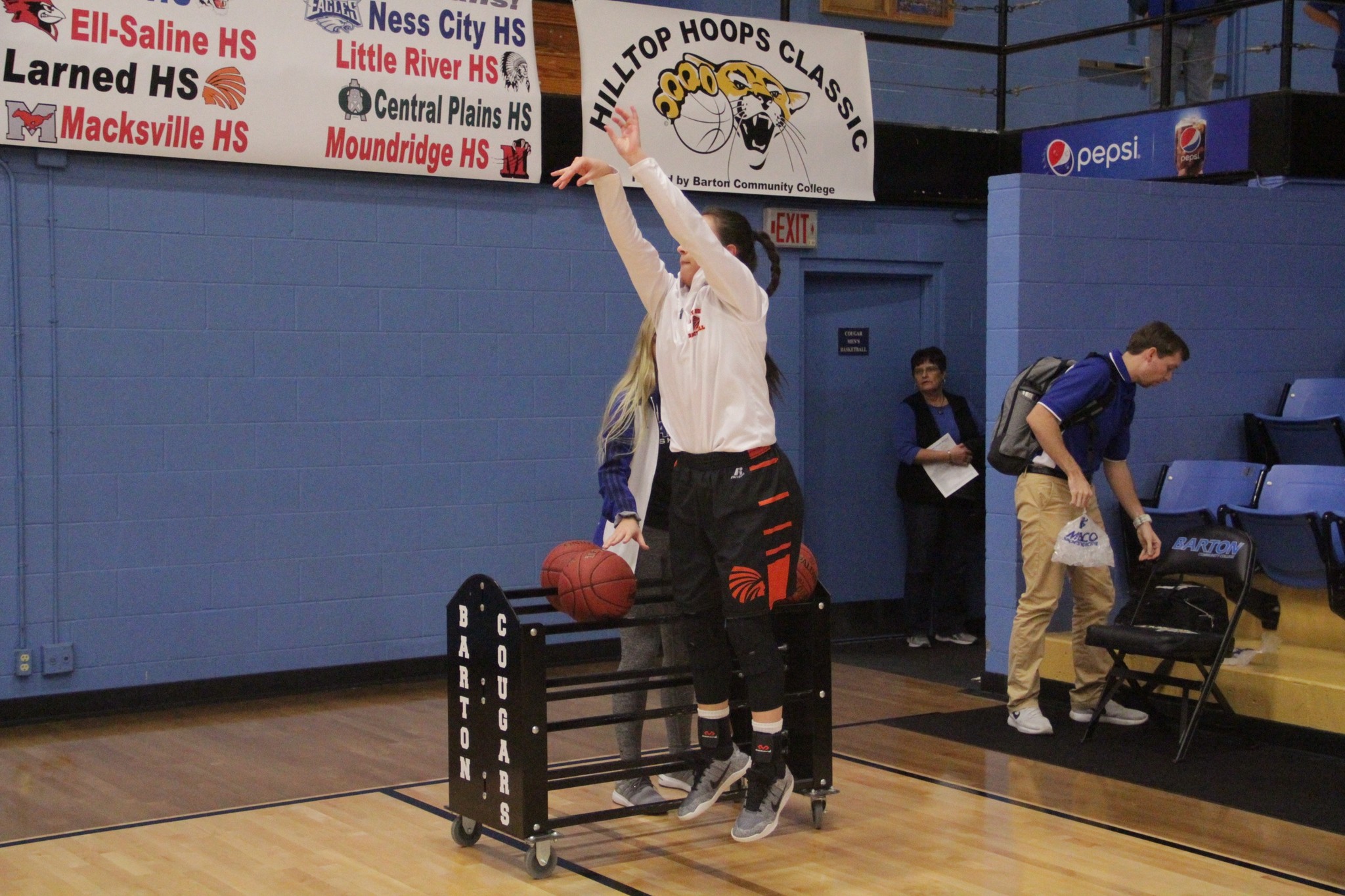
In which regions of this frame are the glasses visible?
[912,367,939,373]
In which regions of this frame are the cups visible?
[1175,120,1207,177]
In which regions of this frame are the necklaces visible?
[924,393,945,415]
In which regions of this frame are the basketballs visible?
[775,542,817,605]
[557,549,637,623]
[539,538,603,613]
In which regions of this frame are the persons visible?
[594,314,699,813]
[551,108,806,842]
[1127,0,1242,108]
[1302,0,1345,94]
[1008,322,1190,735]
[890,346,982,648]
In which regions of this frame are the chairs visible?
[1081,379,1345,764]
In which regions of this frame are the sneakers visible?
[1007,705,1053,735]
[907,635,932,648]
[936,632,977,645]
[612,774,665,809]
[677,741,752,821]
[658,766,696,792]
[731,761,795,842]
[1069,698,1149,726]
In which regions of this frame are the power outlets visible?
[15,649,32,676]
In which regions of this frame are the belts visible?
[1022,466,1093,482]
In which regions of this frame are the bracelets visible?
[1132,513,1152,529]
[947,450,952,464]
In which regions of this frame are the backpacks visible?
[987,351,1118,476]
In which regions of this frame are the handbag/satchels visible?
[1117,580,1228,634]
[1050,505,1114,567]
